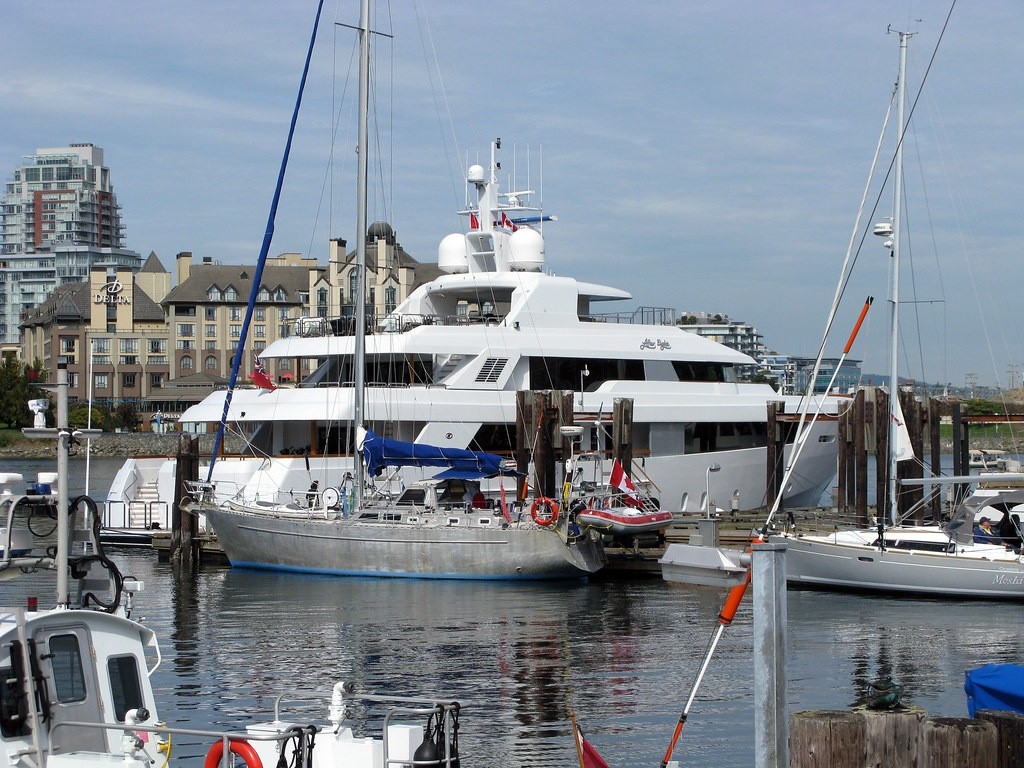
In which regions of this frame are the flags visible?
[253,353,277,390]
[502,212,517,233]
[610,457,644,508]
[470,213,479,229]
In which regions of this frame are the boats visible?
[0,353,463,768]
[96,455,214,546]
[177,0,860,581]
[768,20,1024,599]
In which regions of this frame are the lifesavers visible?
[530,495,558,527]
[204,737,263,768]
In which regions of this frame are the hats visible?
[981,517,991,523]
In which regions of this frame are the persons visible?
[972,514,1023,553]
[306,483,319,508]
